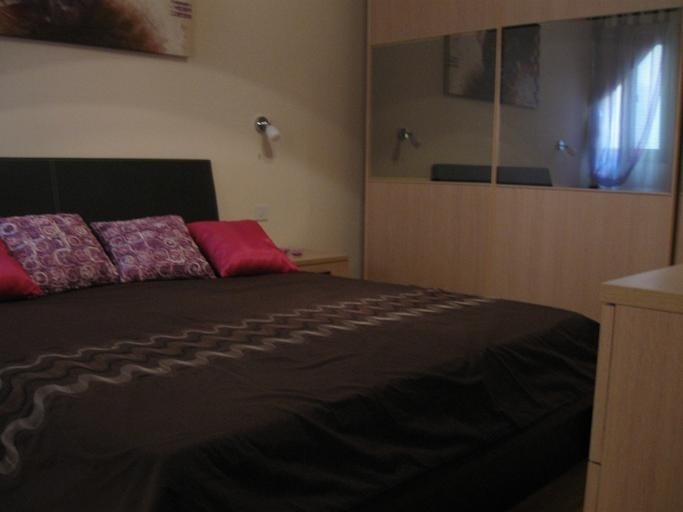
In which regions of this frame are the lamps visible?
[252,115,283,143]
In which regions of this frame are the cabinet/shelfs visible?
[581,266,681,510]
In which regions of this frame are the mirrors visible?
[370,26,497,186]
[496,6,681,198]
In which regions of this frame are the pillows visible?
[186,217,302,278]
[1,214,117,298]
[92,214,218,284]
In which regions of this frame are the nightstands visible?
[292,249,349,277]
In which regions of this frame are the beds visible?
[1,151,595,511]
